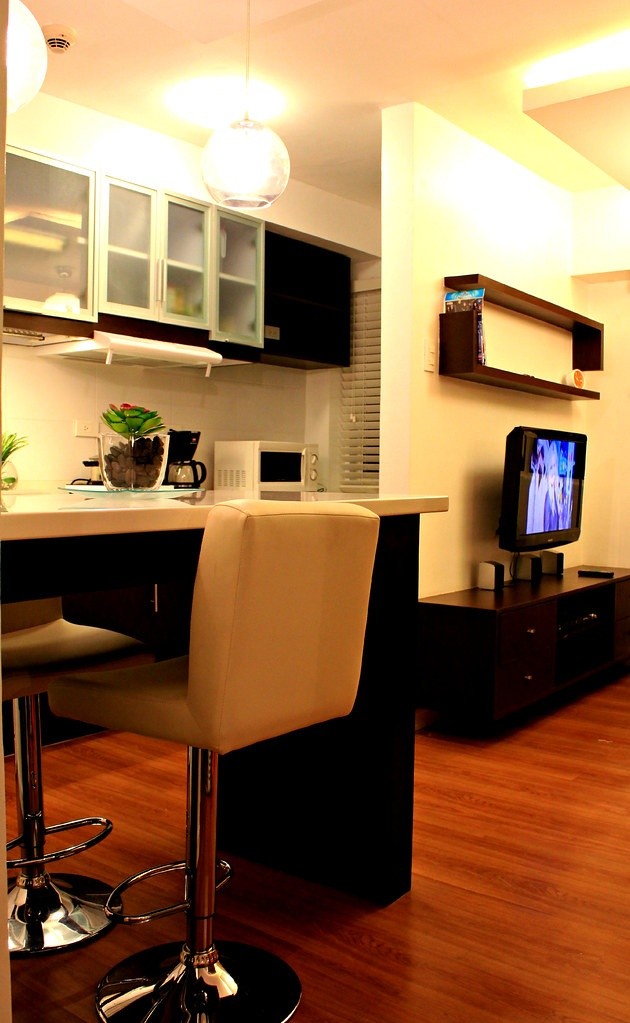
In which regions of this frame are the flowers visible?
[101,401,166,432]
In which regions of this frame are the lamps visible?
[7,0,48,118]
[198,0,290,211]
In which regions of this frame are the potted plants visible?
[1,429,33,490]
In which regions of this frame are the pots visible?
[83,455,103,485]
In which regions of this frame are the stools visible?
[0,589,155,959]
[38,496,382,1023]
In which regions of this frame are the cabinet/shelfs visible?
[417,564,630,739]
[1,142,355,372]
[437,268,609,401]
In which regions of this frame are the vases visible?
[97,428,170,492]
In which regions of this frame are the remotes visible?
[577,570,614,578]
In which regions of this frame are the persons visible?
[543,442,560,531]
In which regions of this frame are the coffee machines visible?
[162,430,207,490]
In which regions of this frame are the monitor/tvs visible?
[499,427,588,554]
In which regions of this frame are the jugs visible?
[167,458,206,489]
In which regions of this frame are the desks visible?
[0,485,453,910]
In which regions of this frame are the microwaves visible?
[214,436,320,497]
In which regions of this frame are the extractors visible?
[33,330,223,378]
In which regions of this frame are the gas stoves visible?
[58,476,204,492]
